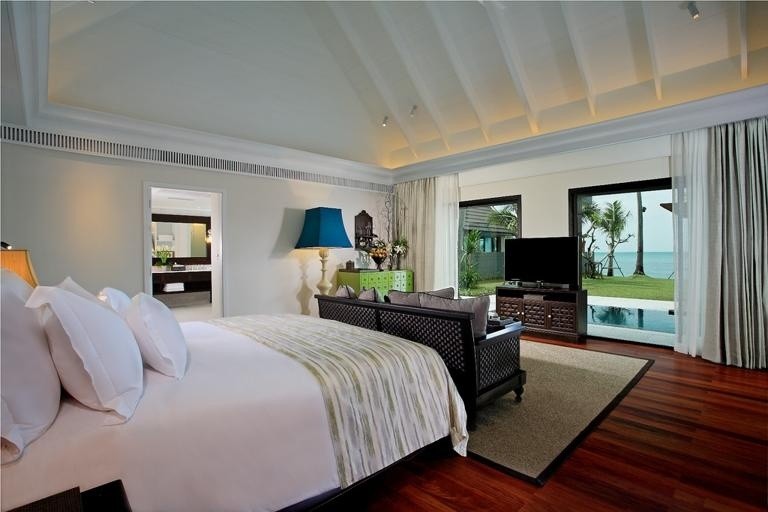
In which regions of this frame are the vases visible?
[396,254,402,270]
[370,256,387,272]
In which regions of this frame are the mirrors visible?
[151,222,206,258]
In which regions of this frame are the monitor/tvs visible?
[503,236,582,291]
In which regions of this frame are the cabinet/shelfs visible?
[152,270,212,304]
[495,285,587,344]
[336,268,413,303]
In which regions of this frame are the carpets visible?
[464,337,656,489]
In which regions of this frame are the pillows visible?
[97,286,129,319]
[334,283,356,299]
[388,287,454,307]
[357,287,383,303]
[417,291,489,339]
[123,291,188,382]
[0,267,61,466]
[22,275,143,427]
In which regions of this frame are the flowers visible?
[388,235,410,260]
[359,240,392,257]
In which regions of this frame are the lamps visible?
[294,207,353,295]
[0,241,40,289]
[207,228,211,237]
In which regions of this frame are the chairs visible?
[584,262,602,280]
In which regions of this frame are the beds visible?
[0,313,468,512]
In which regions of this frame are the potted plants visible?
[151,245,175,272]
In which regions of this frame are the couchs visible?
[313,295,526,431]
